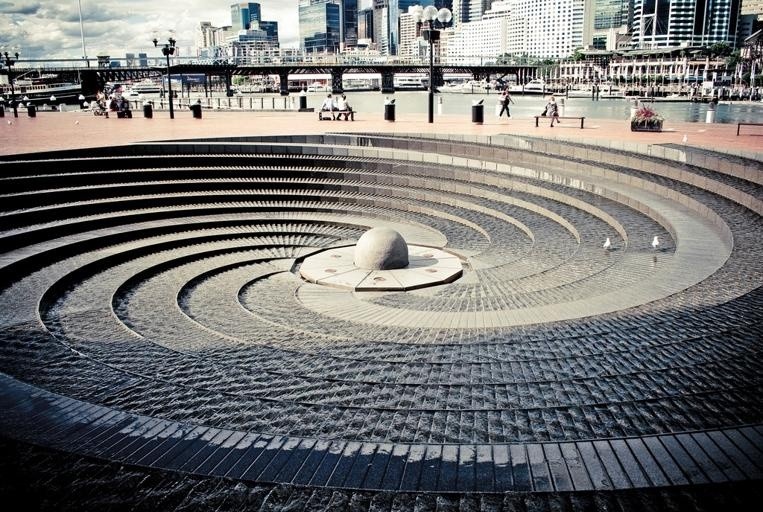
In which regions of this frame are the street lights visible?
[0,50,22,117]
[411,4,453,123]
[152,38,178,120]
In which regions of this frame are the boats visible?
[129,80,167,92]
[0,70,81,105]
[446,78,551,95]
[128,91,139,96]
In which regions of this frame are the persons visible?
[321,94,342,121]
[498,85,516,120]
[544,96,561,128]
[95,89,108,115]
[338,94,355,121]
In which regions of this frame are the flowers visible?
[631,106,664,128]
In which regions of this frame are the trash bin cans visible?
[385,99,395,122]
[193,98,202,119]
[472,98,485,125]
[143,100,152,117]
[0,105,4,117]
[28,104,35,117]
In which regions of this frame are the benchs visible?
[534,117,585,129]
[737,123,763,135]
[316,109,357,121]
